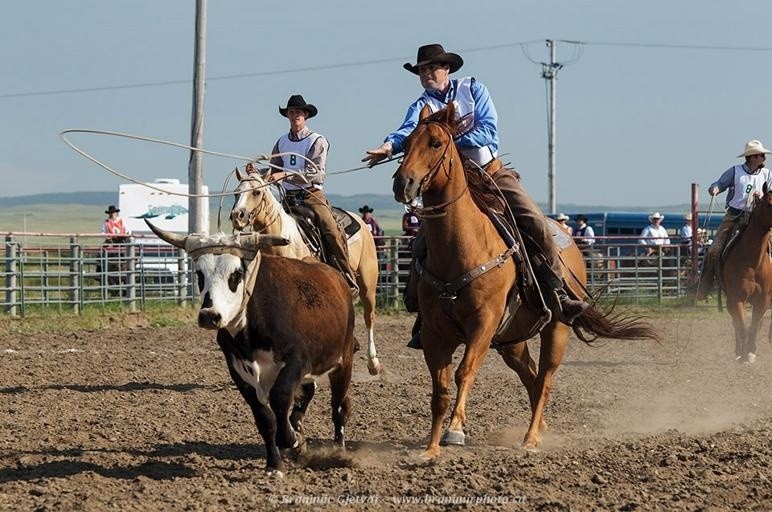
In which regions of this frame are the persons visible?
[680,212,714,259]
[95,203,132,280]
[555,212,574,246]
[637,212,673,255]
[269,93,359,303]
[568,214,596,258]
[400,201,423,233]
[358,204,382,240]
[695,141,772,299]
[360,44,590,350]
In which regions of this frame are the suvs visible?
[96,178,210,297]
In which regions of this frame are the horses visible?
[229,166,380,376]
[716,181,772,368]
[96,229,138,296]
[392,100,666,464]
[620,239,691,294]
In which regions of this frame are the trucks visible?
[545,212,723,266]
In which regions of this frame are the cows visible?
[144,218,355,480]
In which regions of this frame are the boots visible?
[550,293,588,325]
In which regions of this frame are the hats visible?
[105,206,119,213]
[574,215,587,223]
[403,44,463,74]
[684,213,693,220]
[555,213,569,222]
[737,140,772,158]
[279,95,317,119]
[649,213,664,223]
[359,206,373,213]
